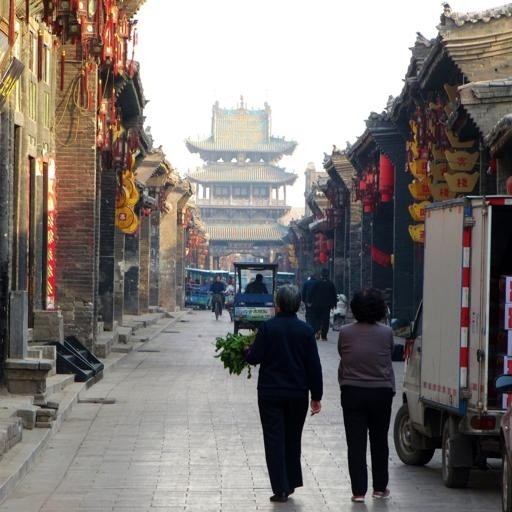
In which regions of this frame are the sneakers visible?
[352,495,365,502]
[373,489,390,498]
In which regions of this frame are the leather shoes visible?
[270,492,287,502]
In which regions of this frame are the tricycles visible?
[186,262,296,335]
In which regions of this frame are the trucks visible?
[392,195,512,489]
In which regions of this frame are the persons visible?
[243,284,323,503]
[226,279,236,323]
[246,274,268,308]
[337,287,396,501]
[313,268,336,340]
[302,274,316,330]
[209,275,224,317]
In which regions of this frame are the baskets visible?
[213,294,222,302]
